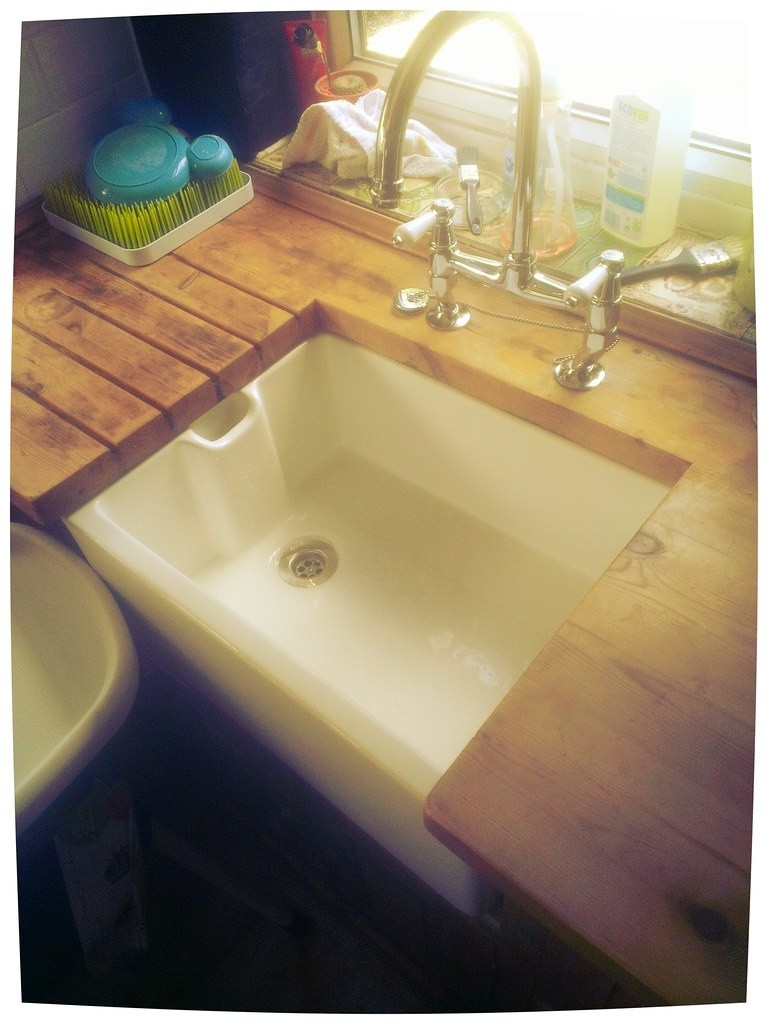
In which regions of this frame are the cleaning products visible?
[504,67,576,259]
[598,85,685,248]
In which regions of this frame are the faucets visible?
[368,11,540,287]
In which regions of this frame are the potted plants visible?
[315,68,380,105]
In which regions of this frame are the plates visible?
[85,122,190,208]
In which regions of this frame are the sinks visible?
[11,528,118,833]
[64,334,672,798]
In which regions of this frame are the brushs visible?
[456,145,484,236]
[618,235,745,286]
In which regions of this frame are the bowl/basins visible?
[122,96,172,126]
[187,134,233,178]
[435,170,504,228]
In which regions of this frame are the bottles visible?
[602,68,692,247]
[501,87,576,259]
